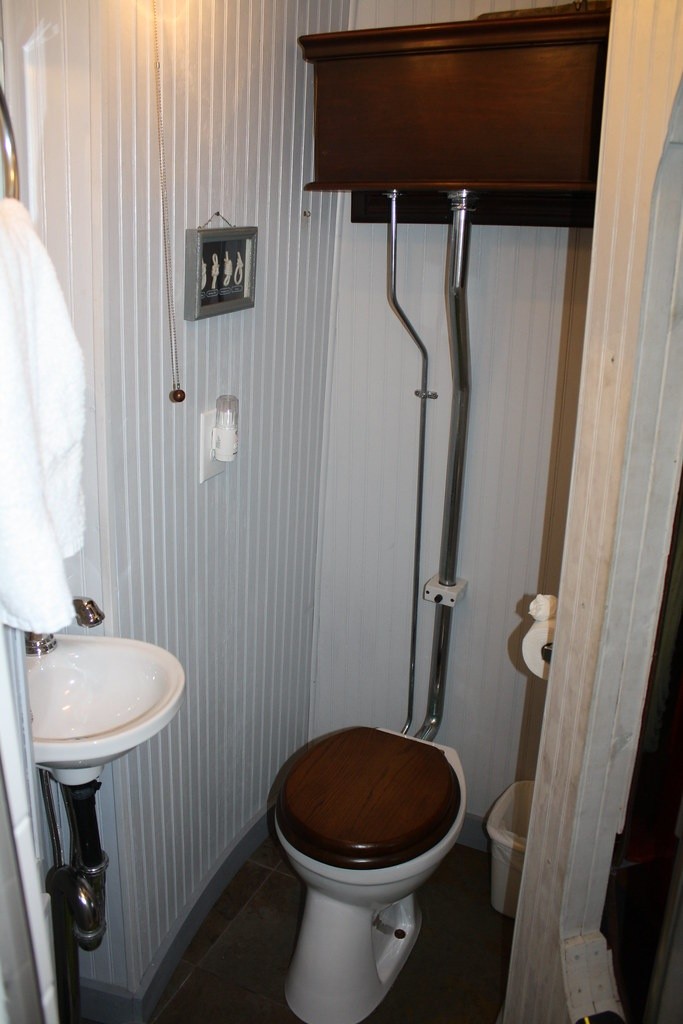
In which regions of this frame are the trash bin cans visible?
[485,780,536,919]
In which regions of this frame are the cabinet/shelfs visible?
[297,4,610,194]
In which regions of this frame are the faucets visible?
[28,597,105,654]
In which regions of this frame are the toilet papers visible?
[523,592,560,679]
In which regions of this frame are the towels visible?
[0,199,87,638]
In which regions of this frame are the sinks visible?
[24,634,185,786]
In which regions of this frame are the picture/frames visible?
[184,225,258,321]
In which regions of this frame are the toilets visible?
[271,722,468,1024]
